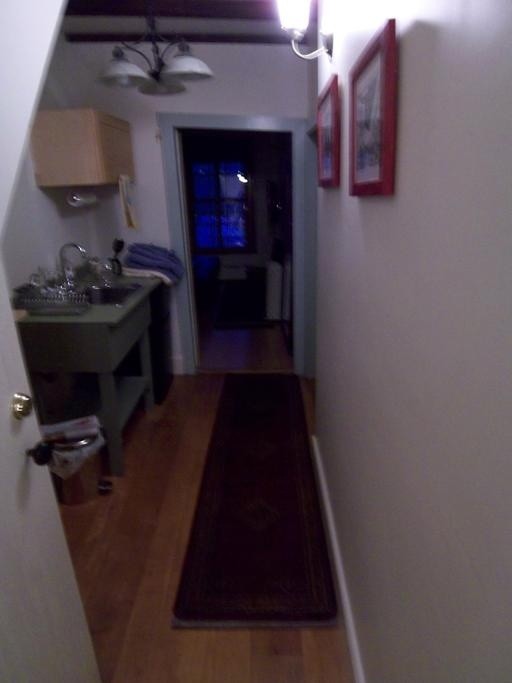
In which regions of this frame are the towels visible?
[116,172,141,228]
[121,243,186,287]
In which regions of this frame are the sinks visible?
[82,283,143,305]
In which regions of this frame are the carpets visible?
[172,371,342,628]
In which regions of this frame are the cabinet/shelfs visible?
[31,108,135,188]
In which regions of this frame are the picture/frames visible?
[348,18,398,197]
[316,70,341,192]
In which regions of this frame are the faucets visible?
[58,241,87,274]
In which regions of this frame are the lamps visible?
[94,0,215,95]
[275,0,333,60]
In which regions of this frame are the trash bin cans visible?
[47,431,105,504]
